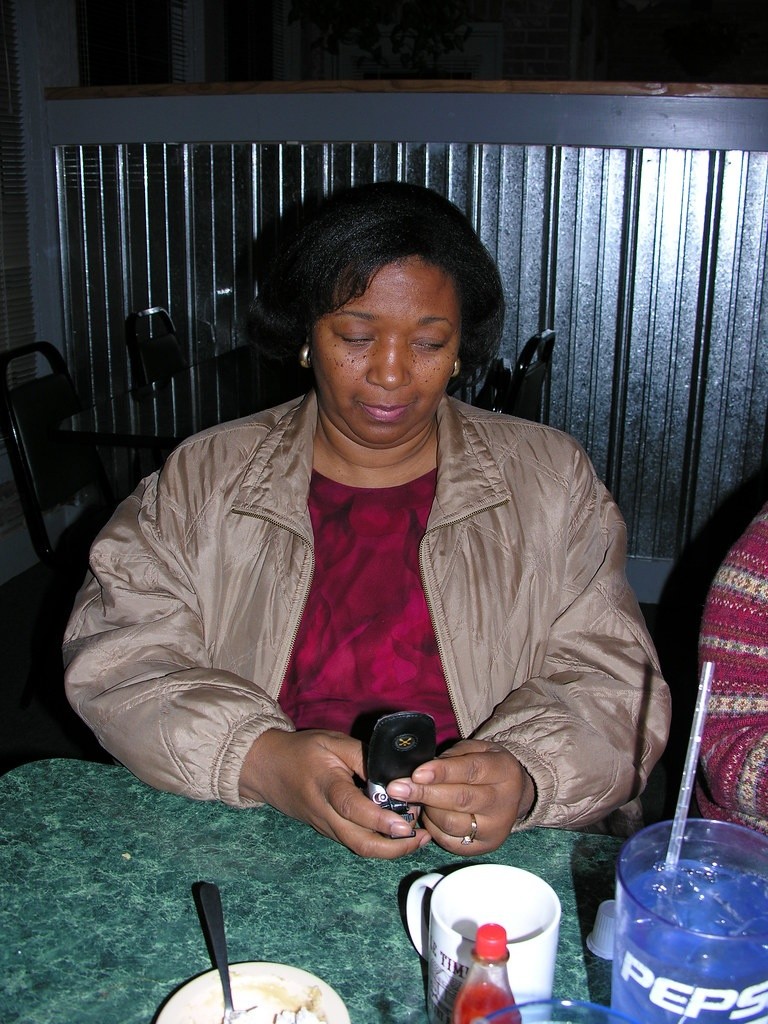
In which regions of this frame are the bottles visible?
[451,923,522,1024]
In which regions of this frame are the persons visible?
[696,503,768,872]
[60,181,673,860]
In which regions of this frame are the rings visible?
[461,814,477,845]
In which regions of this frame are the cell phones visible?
[363,712,434,814]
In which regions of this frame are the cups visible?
[610,818,768,1024]
[406,863,562,1024]
[587,900,618,960]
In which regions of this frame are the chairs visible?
[126,307,186,398]
[472,328,556,423]
[0,341,183,710]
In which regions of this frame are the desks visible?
[0,759,631,1024]
[59,344,315,501]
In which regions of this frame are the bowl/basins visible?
[153,962,352,1024]
